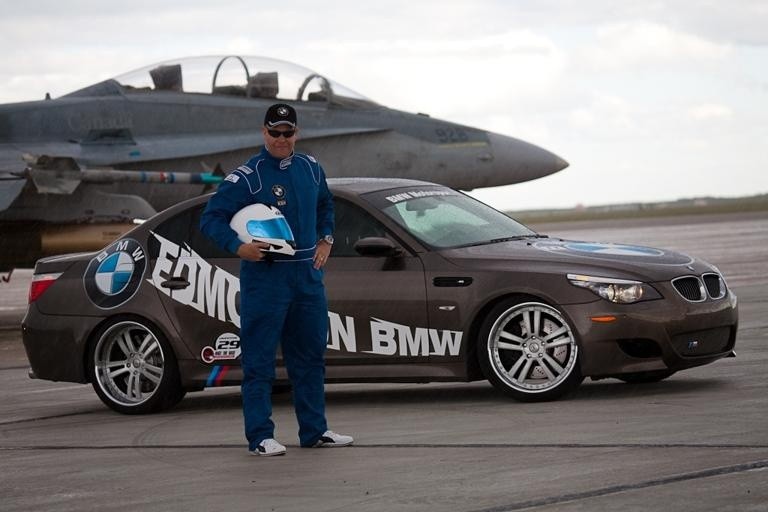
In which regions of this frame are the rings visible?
[321,255,325,258]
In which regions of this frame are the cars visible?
[19,174,738,402]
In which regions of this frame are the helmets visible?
[230,203,297,257]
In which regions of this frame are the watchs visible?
[324,235,334,244]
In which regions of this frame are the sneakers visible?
[249,439,286,456]
[314,431,354,447]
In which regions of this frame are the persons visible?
[199,104,353,456]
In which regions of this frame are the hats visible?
[264,104,296,127]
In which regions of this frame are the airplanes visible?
[0,55,570,286]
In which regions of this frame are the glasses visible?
[268,129,295,138]
